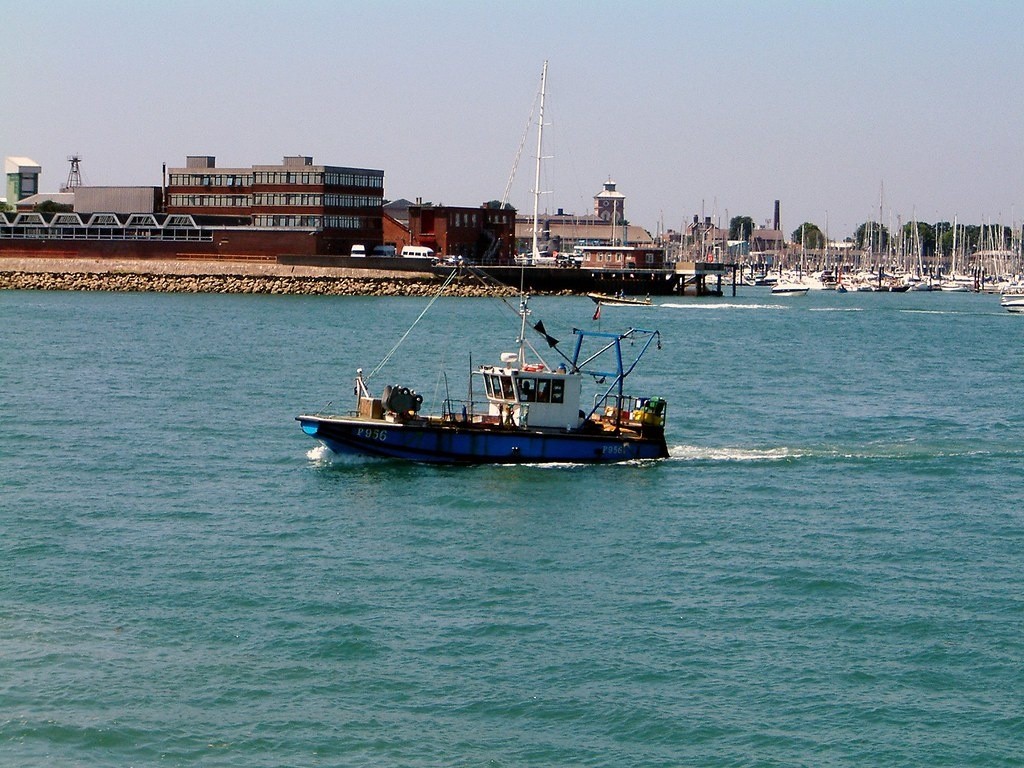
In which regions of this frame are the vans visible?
[370,245,395,256]
[400,245,441,266]
[349,245,367,257]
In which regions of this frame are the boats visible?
[294,256,673,467]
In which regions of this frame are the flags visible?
[593,305,600,320]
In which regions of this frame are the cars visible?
[442,255,478,266]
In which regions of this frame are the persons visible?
[521,381,534,402]
[615,292,619,299]
[621,289,624,299]
[646,293,649,298]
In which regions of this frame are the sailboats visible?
[645,183,1024,316]
[499,59,584,267]
